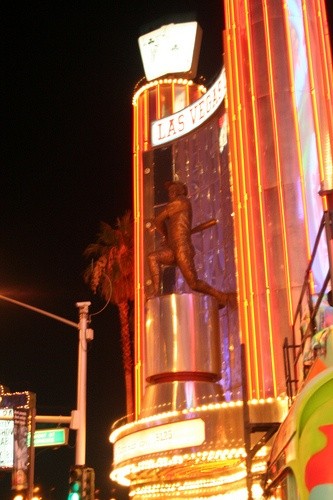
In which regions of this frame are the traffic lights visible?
[68,467,94,500]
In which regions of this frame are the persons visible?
[144,182,228,310]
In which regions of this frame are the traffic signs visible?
[27,426,69,448]
